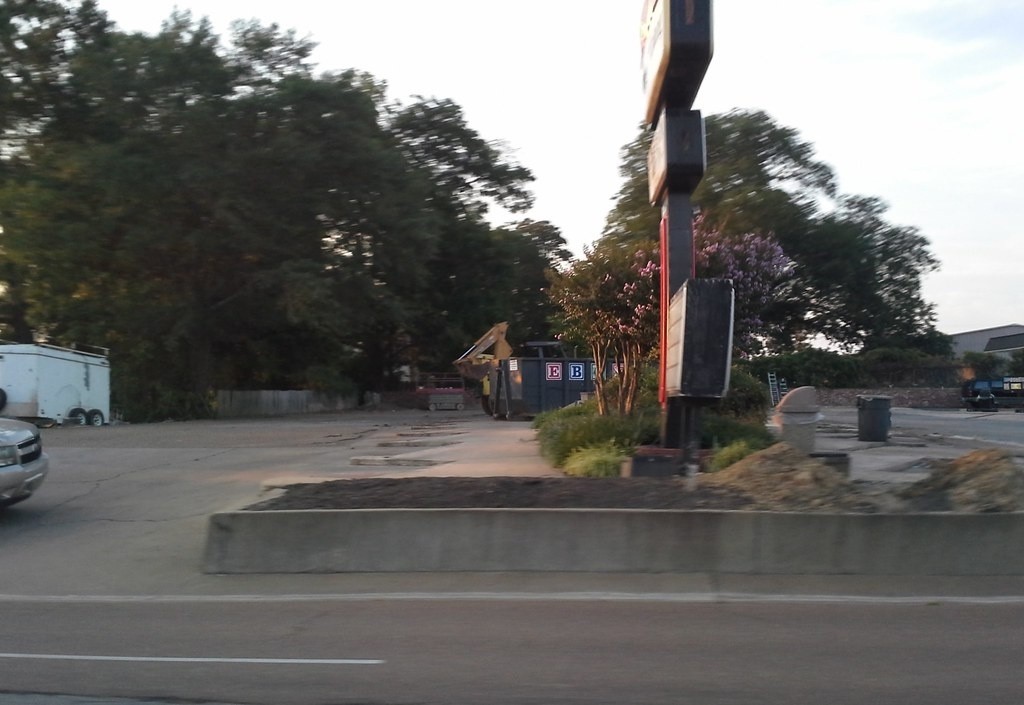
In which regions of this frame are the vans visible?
[959,378,1024,409]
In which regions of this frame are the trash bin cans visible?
[855,394,892,442]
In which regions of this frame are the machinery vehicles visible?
[451,321,567,415]
